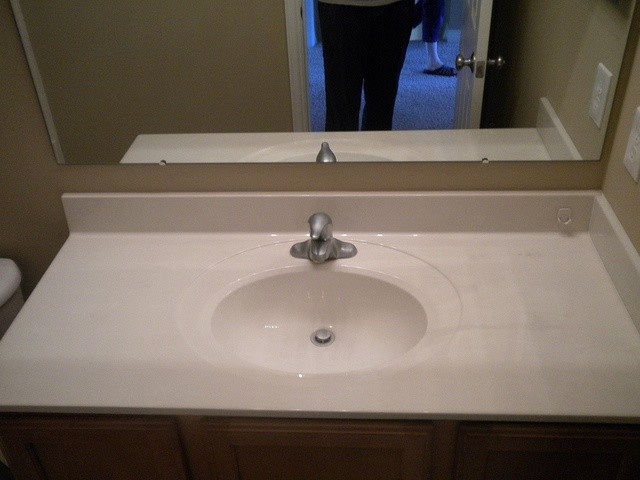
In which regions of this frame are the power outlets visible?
[622,101,640,181]
[586,59,614,131]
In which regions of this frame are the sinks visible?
[175,240,463,378]
[237,139,424,162]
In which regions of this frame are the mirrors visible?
[11,3,637,166]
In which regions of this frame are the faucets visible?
[289,213,359,264]
[315,141,336,163]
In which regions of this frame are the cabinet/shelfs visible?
[1,412,633,479]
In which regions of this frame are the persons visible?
[318,0,422,132]
[422,0,457,76]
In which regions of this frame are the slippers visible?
[422,64,457,75]
